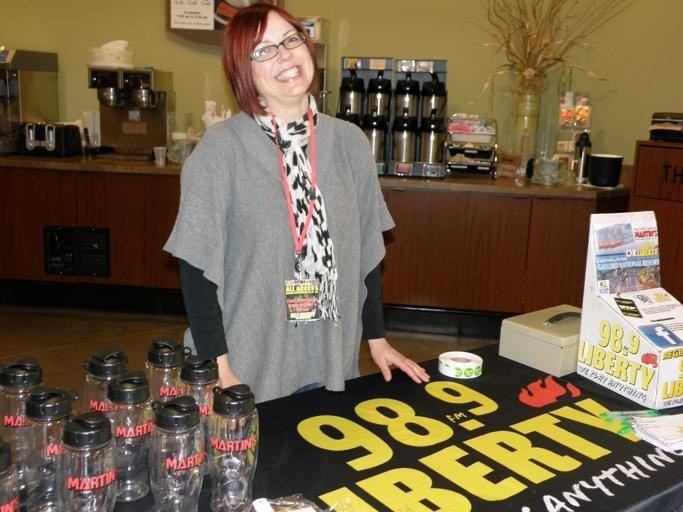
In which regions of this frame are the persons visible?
[162,3,430,405]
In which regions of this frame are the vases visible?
[514,95,540,167]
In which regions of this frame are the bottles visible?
[335,68,448,164]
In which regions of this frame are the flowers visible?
[469,0,634,115]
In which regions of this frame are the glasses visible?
[250,31,308,62]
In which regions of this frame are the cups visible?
[1,440,19,510]
[207,386,260,510]
[3,359,44,470]
[149,396,206,511]
[574,133,592,185]
[80,349,128,414]
[154,147,167,167]
[180,354,220,419]
[59,412,118,511]
[103,371,152,502]
[21,388,79,511]
[145,338,193,401]
[589,154,624,186]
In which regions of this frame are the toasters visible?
[18,121,83,158]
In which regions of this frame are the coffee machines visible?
[85,69,175,162]
[0,48,57,155]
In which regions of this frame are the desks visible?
[1,345,683,512]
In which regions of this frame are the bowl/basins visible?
[88,47,134,68]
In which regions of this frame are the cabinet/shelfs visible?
[629,141,682,304]
[444,116,498,181]
